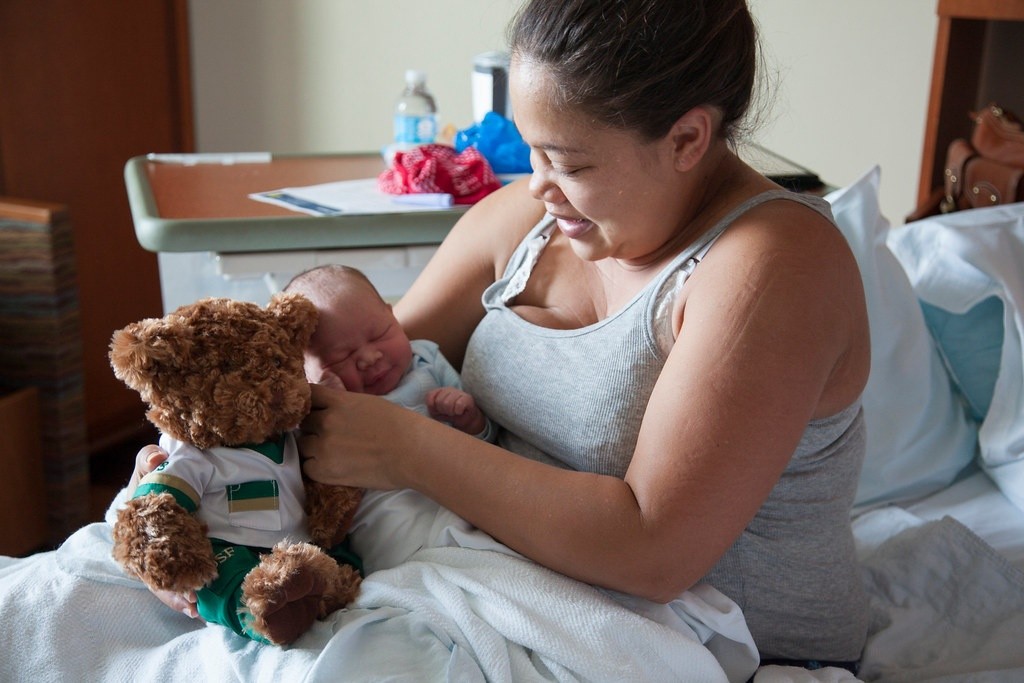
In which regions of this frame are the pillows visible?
[823,165,980,516]
[888,204,1024,519]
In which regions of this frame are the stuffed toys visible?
[112,294,368,646]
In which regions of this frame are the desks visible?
[126,153,498,316]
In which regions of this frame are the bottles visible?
[392,70,436,152]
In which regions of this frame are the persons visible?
[282,266,493,576]
[1,1,874,682]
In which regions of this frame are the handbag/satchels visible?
[941,108,1024,214]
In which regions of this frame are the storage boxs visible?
[0,378,50,558]
